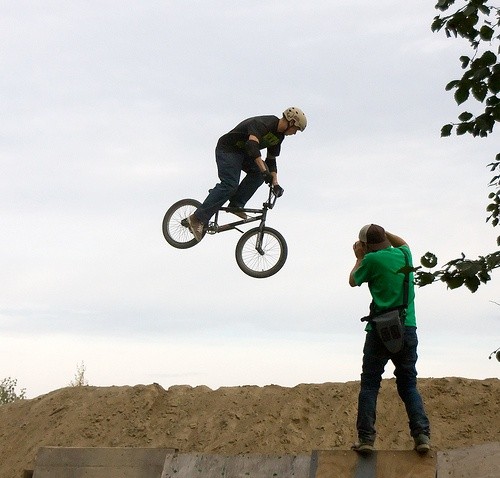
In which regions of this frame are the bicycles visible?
[161,171,288,279]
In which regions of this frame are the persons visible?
[187,106,307,242]
[349,224,431,451]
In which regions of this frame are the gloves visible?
[273,185,284,197]
[262,170,273,187]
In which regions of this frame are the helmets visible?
[283,106,307,132]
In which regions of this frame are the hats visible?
[358,224,385,244]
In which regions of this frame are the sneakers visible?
[187,214,204,242]
[413,434,430,452]
[351,438,375,452]
[230,206,247,219]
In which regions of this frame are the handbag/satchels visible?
[361,304,408,356]
